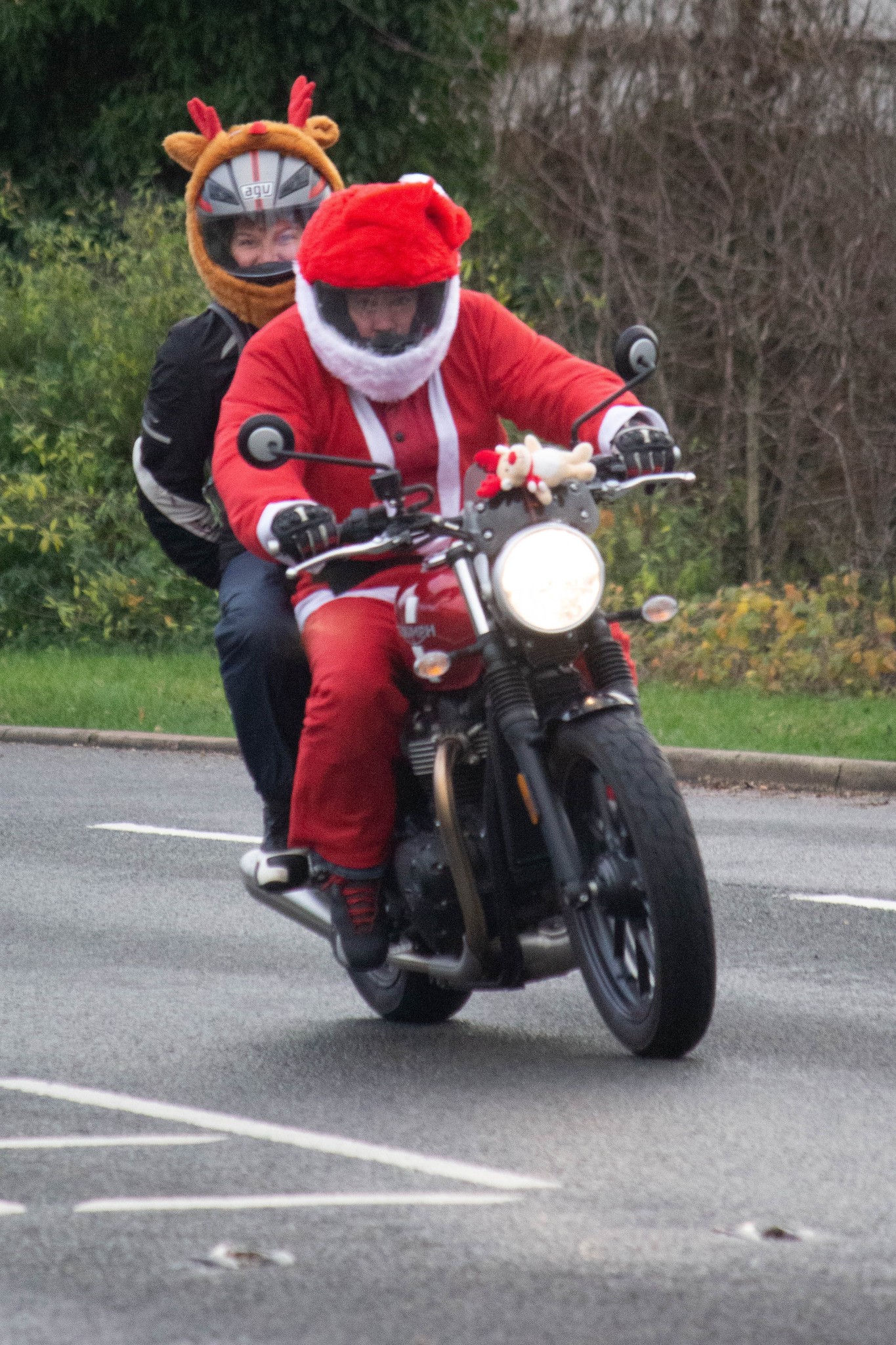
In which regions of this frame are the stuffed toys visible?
[473,434,598,505]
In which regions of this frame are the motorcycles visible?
[227,318,717,1061]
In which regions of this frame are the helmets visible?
[193,149,332,286]
[312,279,445,355]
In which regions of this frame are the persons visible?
[133,76,348,896]
[211,175,676,972]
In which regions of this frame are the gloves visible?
[611,420,674,495]
[270,504,339,563]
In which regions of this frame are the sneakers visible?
[324,865,388,972]
[255,792,309,892]
